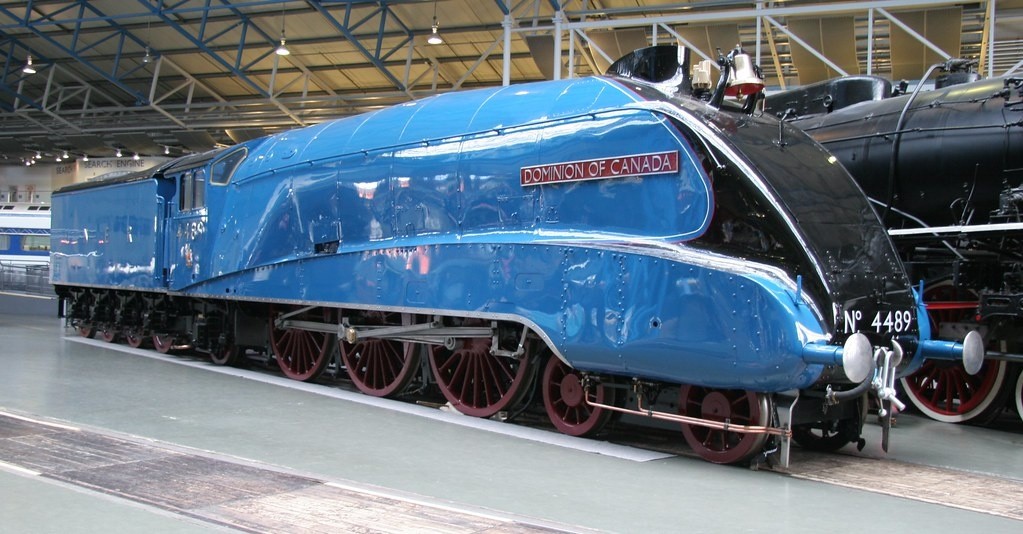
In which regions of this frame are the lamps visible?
[428,1,442,44]
[142,15,154,63]
[22,28,37,73]
[276,3,291,55]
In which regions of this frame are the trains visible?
[762,56,1023,424]
[49,43,983,465]
